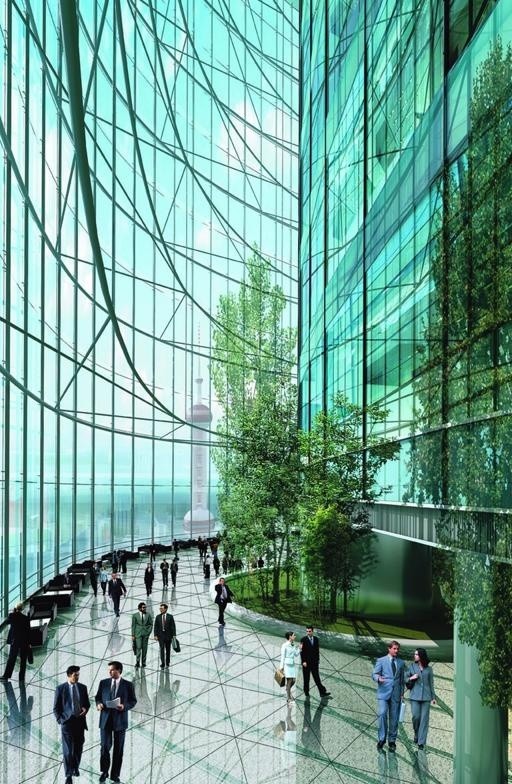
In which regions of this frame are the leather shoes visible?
[110,776,120,782]
[388,742,396,752]
[320,692,331,697]
[377,739,386,749]
[73,767,79,777]
[65,777,72,784]
[99,772,109,782]
[304,691,309,696]
[414,736,418,743]
[419,744,423,750]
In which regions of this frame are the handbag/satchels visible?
[406,663,416,689]
[27,646,34,664]
[399,699,406,722]
[132,641,136,655]
[171,638,181,652]
[274,670,285,687]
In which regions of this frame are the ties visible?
[111,680,116,701]
[391,658,396,675]
[72,684,80,720]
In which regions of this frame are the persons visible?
[279,631,304,703]
[94,661,137,782]
[54,665,91,784]
[195,528,264,579]
[403,648,437,752]
[214,577,234,626]
[299,625,331,697]
[88,537,180,668]
[0,602,32,681]
[371,640,404,754]
